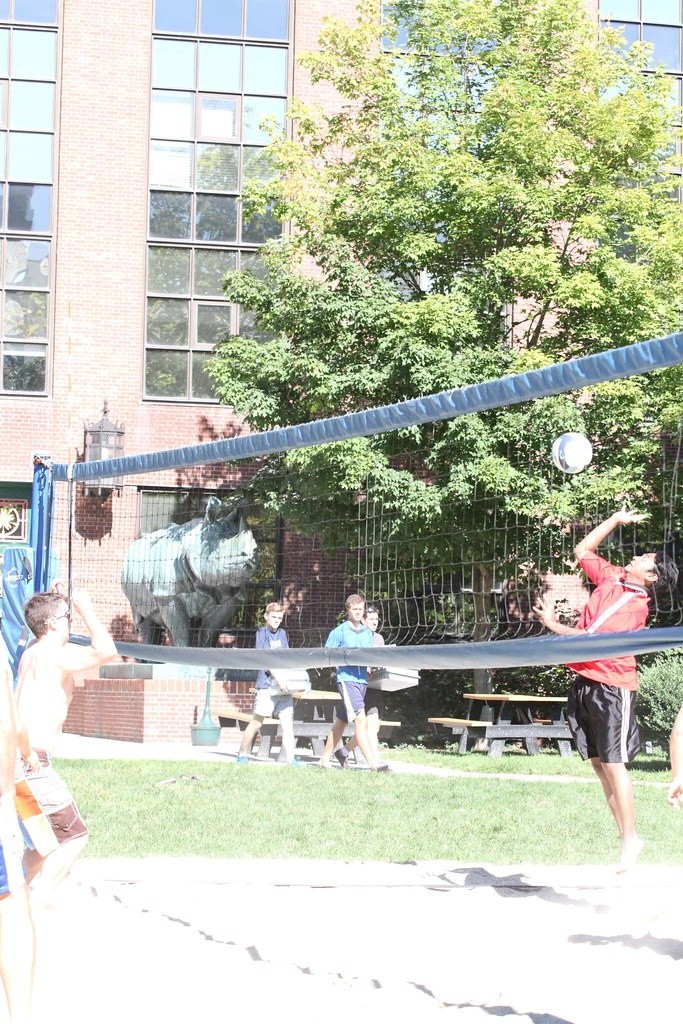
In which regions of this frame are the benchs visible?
[429,718,579,757]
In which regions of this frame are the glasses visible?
[47,611,70,622]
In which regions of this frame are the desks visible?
[246,687,342,759]
[457,694,567,754]
[211,709,401,762]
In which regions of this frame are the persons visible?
[532,504,677,874]
[236,602,300,765]
[14,583,117,910]
[667,702,683,810]
[0,631,37,1024]
[317,594,389,772]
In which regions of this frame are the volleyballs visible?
[551,432,594,475]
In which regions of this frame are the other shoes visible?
[291,760,303,768]
[333,748,349,768]
[371,763,389,771]
[319,758,333,769]
[238,755,249,764]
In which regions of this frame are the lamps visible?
[81,400,125,496]
